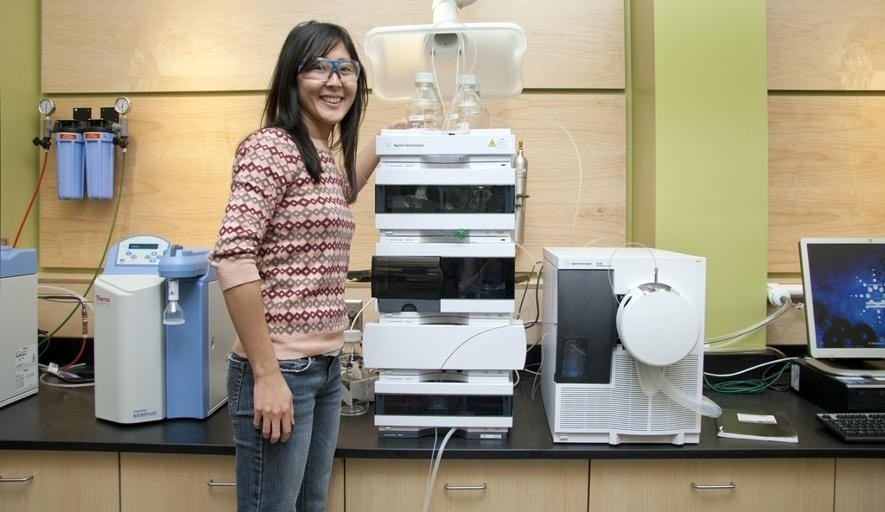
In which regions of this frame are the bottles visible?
[448,72,483,127]
[408,71,445,130]
[337,328,370,417]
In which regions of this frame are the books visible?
[714,406,801,444]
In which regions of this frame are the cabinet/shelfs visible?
[0,450,119,511]
[345,453,589,511]
[119,453,344,512]
[835,455,884,512]
[589,456,837,511]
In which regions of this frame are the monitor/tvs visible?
[799,236,885,377]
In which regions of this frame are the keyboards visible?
[817,411,885,444]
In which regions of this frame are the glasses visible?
[300,57,361,81]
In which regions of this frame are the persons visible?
[207,19,433,511]
